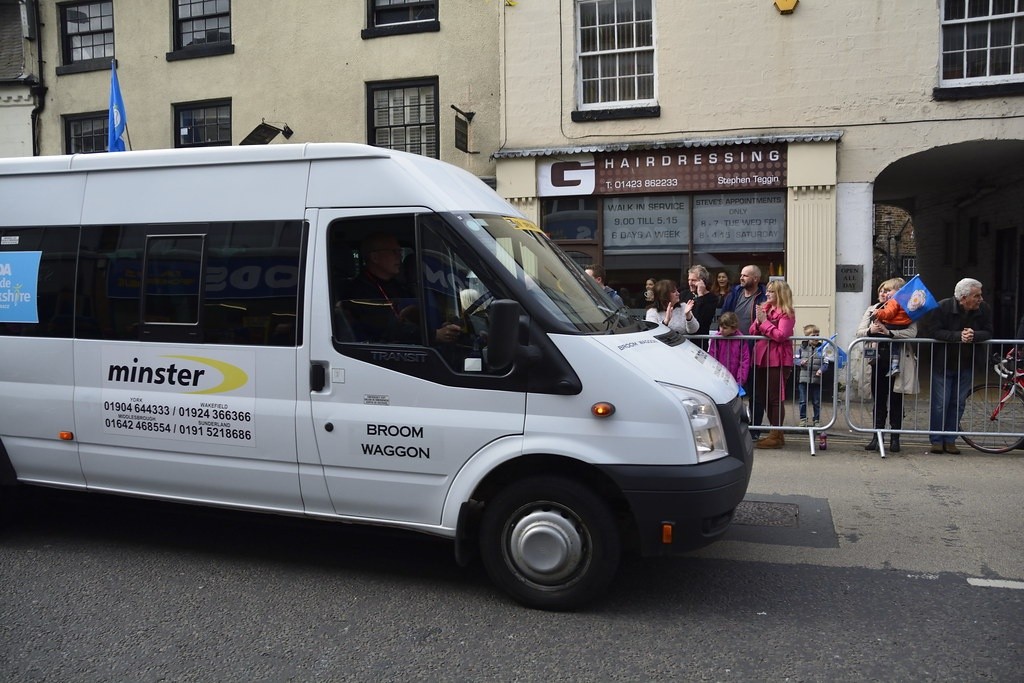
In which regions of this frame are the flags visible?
[107,66,126,152]
[818,336,847,369]
[893,276,938,322]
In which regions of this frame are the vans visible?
[0,143,753,611]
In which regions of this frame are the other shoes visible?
[751,433,759,441]
[799,418,808,427]
[813,419,820,427]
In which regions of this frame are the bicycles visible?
[957,347,1024,454]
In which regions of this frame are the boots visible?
[889,434,900,452]
[865,433,884,450]
[756,430,785,449]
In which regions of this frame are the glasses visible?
[766,290,774,293]
[367,249,403,257]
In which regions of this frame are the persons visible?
[792,325,829,427]
[645,264,795,448]
[585,264,625,314]
[925,278,992,454]
[856,278,921,451]
[353,234,465,348]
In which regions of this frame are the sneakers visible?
[931,444,961,455]
